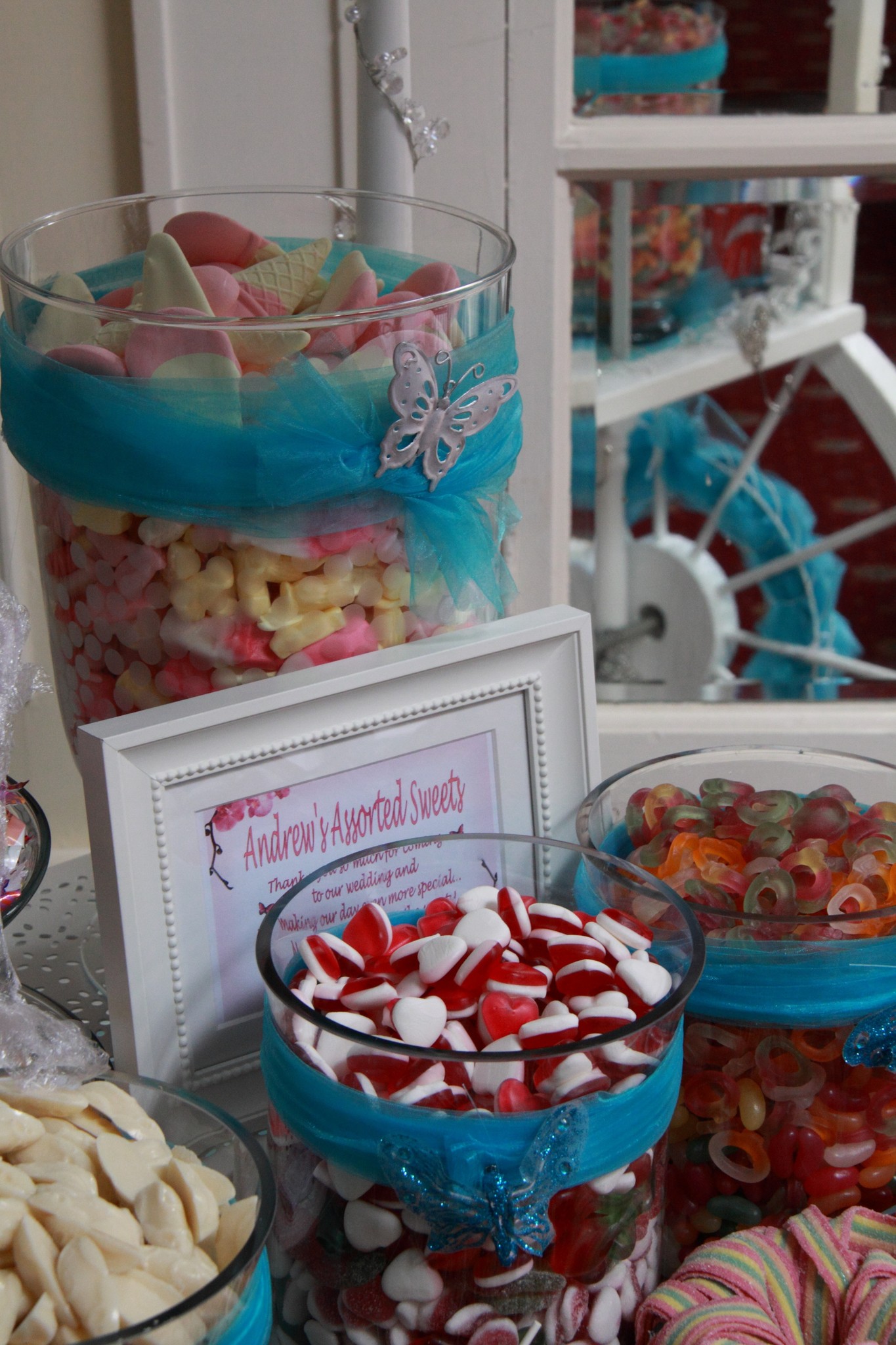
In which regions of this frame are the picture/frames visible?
[73,604,601,1178]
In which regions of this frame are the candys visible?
[2,210,894,1345]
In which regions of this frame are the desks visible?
[0,841,896,1343]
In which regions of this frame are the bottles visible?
[3,175,896,1345]
